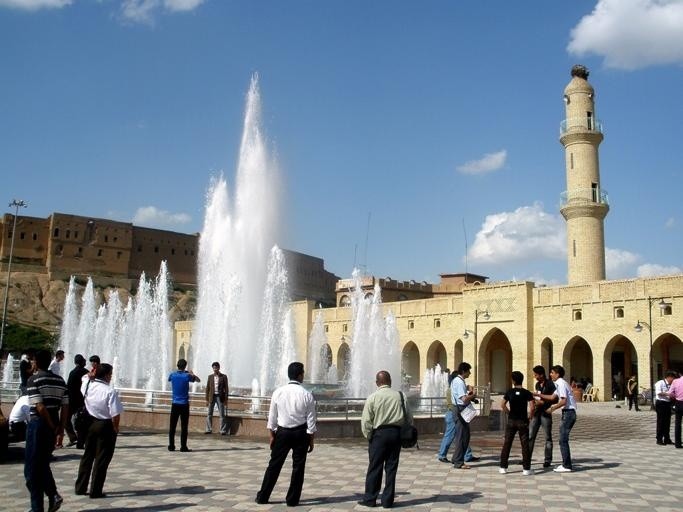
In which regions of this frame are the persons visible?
[168,358,200,451]
[438,362,576,473]
[203,362,228,435]
[358,371,412,506]
[12,346,123,512]
[255,362,316,507]
[584,381,592,394]
[667,366,683,448]
[627,374,642,412]
[655,371,675,445]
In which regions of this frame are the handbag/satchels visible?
[458,403,478,425]
[399,424,418,449]
[73,410,93,433]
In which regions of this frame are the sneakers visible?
[553,465,571,472]
[521,469,534,476]
[219,432,226,435]
[498,467,507,473]
[543,465,554,468]
[203,431,212,435]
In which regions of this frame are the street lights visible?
[0,196,28,348]
[632,294,672,411]
[339,334,352,345]
[461,307,491,395]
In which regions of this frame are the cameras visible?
[468,391,478,404]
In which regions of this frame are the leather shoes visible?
[47,493,63,512]
[65,439,78,447]
[286,503,298,506]
[656,441,666,445]
[663,441,675,445]
[82,492,90,495]
[168,449,175,451]
[89,493,106,498]
[455,464,471,469]
[465,456,480,462]
[438,457,450,462]
[356,500,376,507]
[381,504,393,508]
[180,448,193,452]
[255,498,267,503]
[27,508,32,512]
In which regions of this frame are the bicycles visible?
[636,384,655,406]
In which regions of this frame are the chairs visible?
[581,386,599,402]
